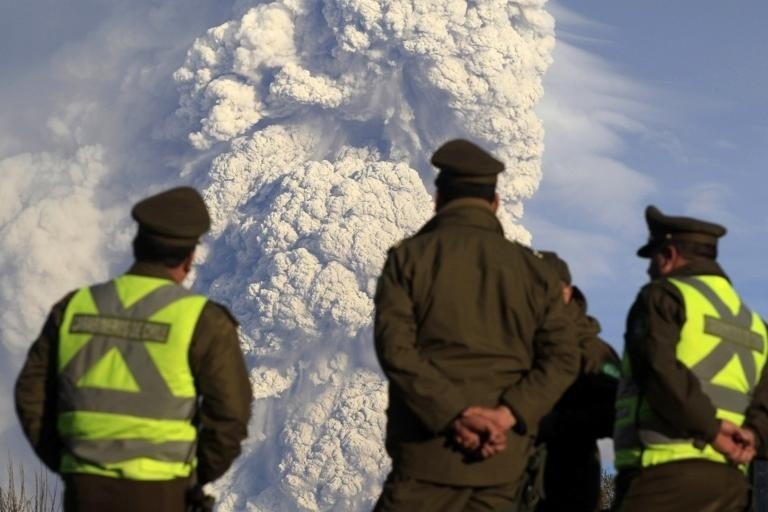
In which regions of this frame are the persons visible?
[373,140,581,511]
[14,187,251,512]
[537,251,622,512]
[610,206,768,512]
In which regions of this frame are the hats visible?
[432,140,503,184]
[637,207,726,257]
[132,187,210,246]
